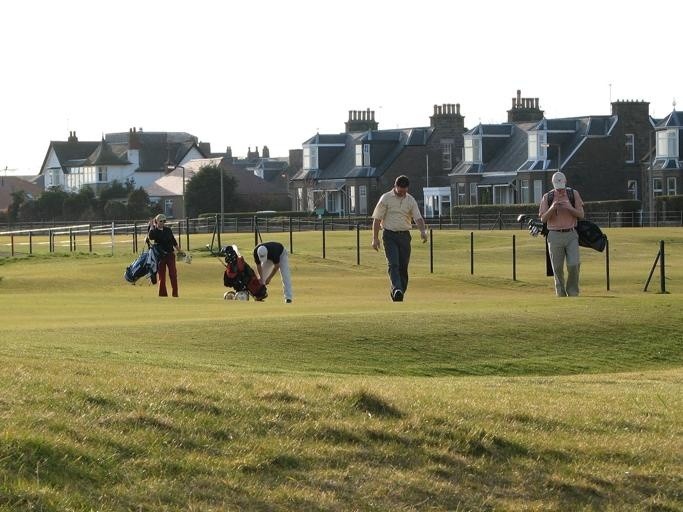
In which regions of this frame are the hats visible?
[156,214,166,220]
[257,245,268,262]
[552,172,566,191]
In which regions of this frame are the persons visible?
[253,241,293,304]
[538,172,584,297]
[145,214,181,298]
[371,175,427,301]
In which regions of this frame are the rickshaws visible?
[220,245,269,301]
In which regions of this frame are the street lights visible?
[168,164,185,234]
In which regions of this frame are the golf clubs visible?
[517,214,544,237]
[206,244,237,268]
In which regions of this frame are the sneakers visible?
[286,299,291,303]
[392,288,403,302]
